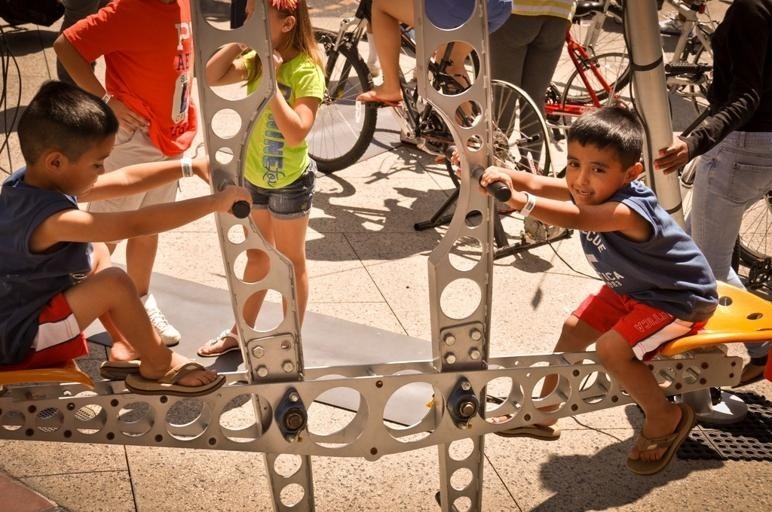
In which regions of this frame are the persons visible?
[197,1,329,384]
[652,0,771,390]
[0,81,252,399]
[54,1,200,347]
[56,1,114,89]
[449,107,720,476]
[354,1,517,163]
[487,1,578,178]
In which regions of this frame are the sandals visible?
[729,359,772,388]
[197,328,242,356]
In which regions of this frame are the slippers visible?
[625,400,694,477]
[490,413,561,441]
[356,90,404,108]
[99,355,141,379]
[125,363,225,396]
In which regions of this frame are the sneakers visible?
[658,16,696,39]
[135,291,181,346]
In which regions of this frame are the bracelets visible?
[98,92,113,106]
[177,156,197,183]
[518,189,537,222]
